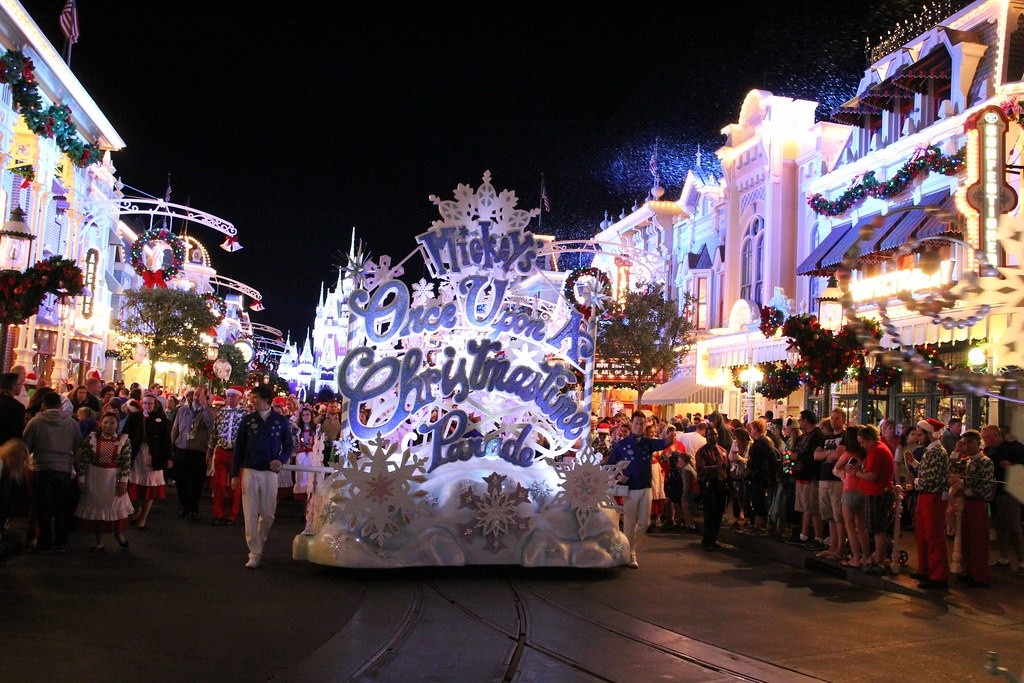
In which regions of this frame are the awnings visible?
[796,189,966,277]
[632,377,723,405]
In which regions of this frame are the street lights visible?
[0,204,37,374]
[207,342,219,392]
[815,272,845,418]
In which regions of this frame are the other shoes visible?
[114,534,128,548]
[212,517,236,526]
[629,551,639,568]
[1016,566,1024,576]
[990,560,1011,572]
[737,524,991,596]
[702,540,721,551]
[37,545,67,554]
[89,545,105,553]
[245,556,261,568]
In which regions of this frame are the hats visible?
[225,385,244,397]
[212,395,225,405]
[151,383,163,389]
[917,417,944,432]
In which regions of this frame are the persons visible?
[229,385,293,569]
[591,408,1024,592]
[0,366,344,550]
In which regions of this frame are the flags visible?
[59,0,79,44]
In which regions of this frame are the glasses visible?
[16,384,23,386]
